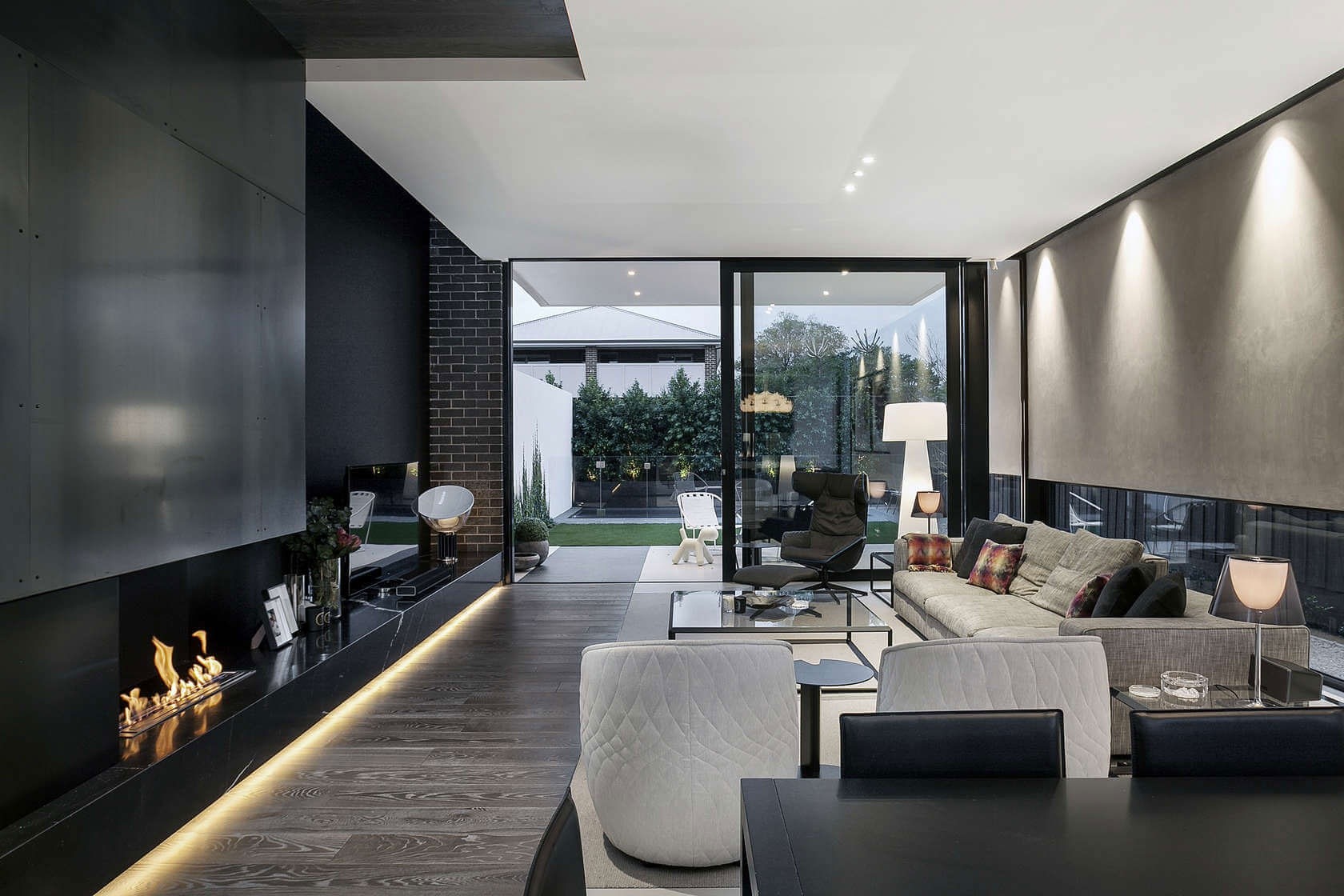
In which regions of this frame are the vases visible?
[321,557,342,620]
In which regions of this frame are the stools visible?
[732,565,823,621]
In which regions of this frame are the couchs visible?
[872,634,1114,778]
[891,536,1311,757]
[578,636,797,868]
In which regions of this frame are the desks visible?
[736,775,1344,896]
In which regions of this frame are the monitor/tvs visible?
[343,462,421,600]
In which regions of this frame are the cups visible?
[285,558,341,632]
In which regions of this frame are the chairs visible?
[522,785,590,896]
[838,706,1069,783]
[778,470,870,605]
[1126,706,1344,779]
[673,471,709,506]
[676,492,744,558]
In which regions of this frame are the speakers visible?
[1247,654,1322,708]
[396,566,451,596]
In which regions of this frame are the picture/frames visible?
[258,595,293,650]
[261,582,299,635]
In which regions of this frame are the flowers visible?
[279,495,362,562]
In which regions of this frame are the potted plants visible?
[514,517,550,566]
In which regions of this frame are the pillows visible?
[1027,528,1144,618]
[951,517,1013,573]
[1090,560,1157,618]
[1064,572,1112,620]
[993,513,1032,530]
[1123,569,1186,618]
[1005,520,1076,602]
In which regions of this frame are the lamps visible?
[910,491,950,535]
[956,521,1028,580]
[1207,553,1307,709]
[964,538,1024,596]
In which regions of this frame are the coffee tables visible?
[668,590,894,694]
[791,658,874,779]
[870,551,895,609]
[732,542,780,595]
[1107,684,1344,779]
